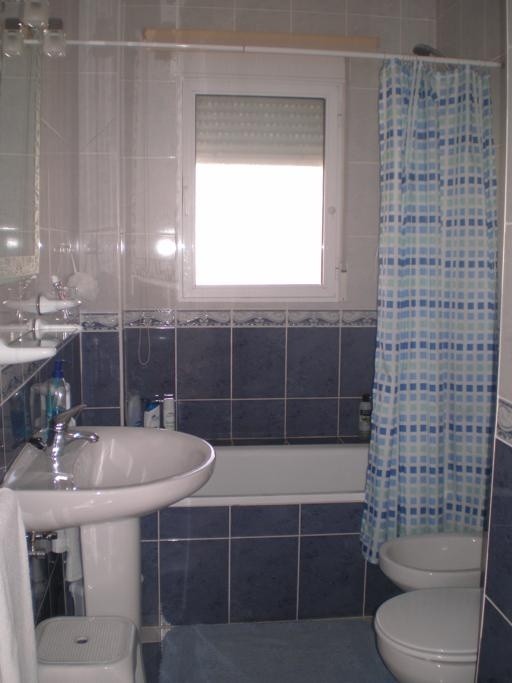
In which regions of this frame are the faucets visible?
[45,401,99,472]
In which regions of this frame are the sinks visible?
[0,425,216,532]
[378,532,488,586]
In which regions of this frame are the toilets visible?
[374,586,485,683]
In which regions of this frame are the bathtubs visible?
[139,443,373,632]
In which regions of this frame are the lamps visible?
[3,14,39,58]
[38,14,64,58]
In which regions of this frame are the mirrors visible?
[179,75,338,298]
[0,0,42,281]
